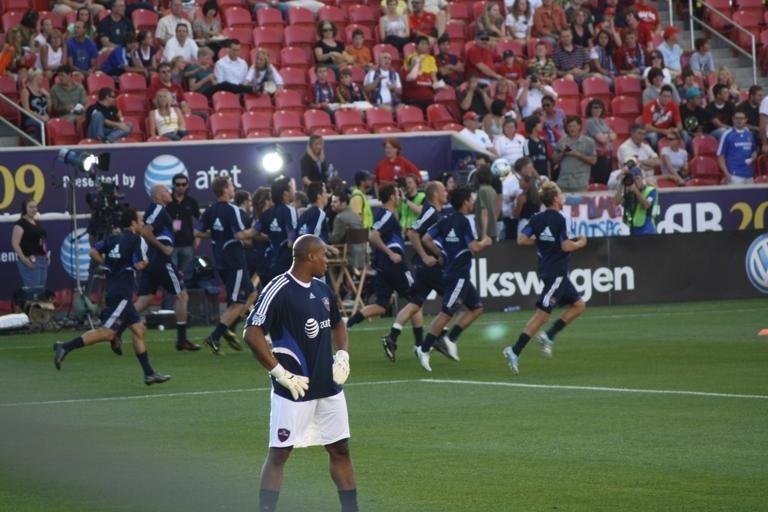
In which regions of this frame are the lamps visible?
[56,148,111,332]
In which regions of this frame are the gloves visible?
[331,349,351,386]
[270,362,310,401]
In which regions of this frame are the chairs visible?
[253,24,284,46]
[318,5,349,25]
[426,103,455,128]
[279,129,308,138]
[114,93,152,116]
[403,43,430,64]
[613,75,647,100]
[303,108,338,133]
[373,125,404,134]
[245,131,274,138]
[580,96,613,119]
[656,179,680,187]
[582,76,614,101]
[311,128,342,136]
[242,91,276,113]
[116,116,144,138]
[657,136,686,156]
[47,118,78,146]
[119,72,148,93]
[111,136,141,144]
[129,9,161,36]
[338,66,367,85]
[182,114,209,136]
[731,8,756,50]
[555,98,580,116]
[284,23,318,46]
[365,106,401,130]
[327,226,372,323]
[334,106,368,130]
[20,76,51,94]
[0,74,22,129]
[344,23,375,46]
[689,156,725,183]
[433,40,462,61]
[52,75,85,89]
[472,0,503,21]
[36,10,64,29]
[211,133,242,140]
[603,116,631,140]
[224,6,258,28]
[372,43,403,66]
[96,50,112,66]
[342,126,372,136]
[77,138,105,145]
[182,91,213,115]
[406,124,436,132]
[685,178,716,187]
[587,183,609,191]
[217,48,250,67]
[692,134,720,161]
[446,2,473,20]
[434,84,461,105]
[287,6,321,26]
[179,134,208,141]
[443,21,465,41]
[737,1,756,11]
[739,91,750,101]
[86,73,120,96]
[162,8,189,34]
[271,108,306,134]
[396,104,431,129]
[704,0,736,42]
[550,78,580,100]
[526,38,553,59]
[464,40,493,63]
[145,136,177,143]
[255,7,288,27]
[348,5,378,25]
[222,26,252,44]
[280,46,311,68]
[278,64,309,89]
[1,9,35,33]
[241,110,273,133]
[674,74,705,92]
[494,38,525,59]
[192,9,222,30]
[273,89,308,111]
[209,111,241,134]
[96,8,113,25]
[439,123,465,131]
[707,71,737,91]
[64,9,95,25]
[211,91,245,114]
[611,95,643,126]
[84,93,101,109]
[249,48,281,70]
[308,66,341,87]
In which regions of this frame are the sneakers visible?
[222,328,242,350]
[502,346,520,376]
[144,372,171,385]
[110,337,122,355]
[380,335,397,362]
[535,331,554,357]
[206,335,220,352]
[442,335,460,361]
[176,340,201,351]
[414,345,433,372]
[53,341,68,370]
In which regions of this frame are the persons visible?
[491,159,522,240]
[378,0,409,44]
[149,88,188,141]
[170,56,189,87]
[675,69,698,99]
[240,50,284,93]
[564,0,594,27]
[704,84,737,141]
[531,109,561,161]
[584,99,616,184]
[759,95,768,159]
[99,32,145,78]
[510,175,540,241]
[83,87,133,145]
[294,191,309,218]
[524,115,548,178]
[460,111,496,157]
[614,168,656,235]
[497,49,525,85]
[679,86,706,152]
[305,63,335,103]
[482,99,507,144]
[540,96,567,138]
[363,53,403,106]
[346,28,371,66]
[608,154,648,190]
[401,35,438,87]
[301,135,340,188]
[12,199,48,288]
[49,0,106,17]
[623,7,655,51]
[243,234,358,512]
[50,64,87,122]
[642,85,682,152]
[85,185,120,299]
[214,39,248,93]
[616,30,645,74]
[145,62,191,120]
[21,67,51,146]
[487,79,518,114]
[34,18,52,52]
[55,208,172,385]
[193,177,257,356]
[533,0,566,38]
[381,180,447,363]
[408,1,450,40]
[657,26,683,72]
[67,8,96,37]
[514,158,544,181]
[434,36,464,88]
[129,29,157,73]
[336,68,365,102]
[244,186,276,288]
[293,181,329,247]
[63,21,97,74]
[374,137,423,186]
[98,0,133,50]
[553,115,597,190]
[570,10,597,49]
[595,6,623,48]
[468,165,503,243]
[457,73,490,117]
[527,42,556,82]
[188,46,219,95]
[689,38,715,81]
[1,28,24,73]
[234,175,297,288]
[408,0,439,45]
[416,185,492,371]
[629,0,659,29]
[160,23,198,66]
[13,8,39,51]
[591,31,618,81]
[596,0,626,28]
[443,173,455,192]
[345,184,424,354]
[517,67,557,118]
[660,132,689,188]
[464,29,514,84]
[350,170,377,232]
[736,86,764,137]
[618,124,659,185]
[717,111,757,185]
[642,50,671,86]
[466,155,491,185]
[493,117,530,167]
[36,30,63,72]
[715,65,741,101]
[112,184,202,355]
[505,0,531,42]
[476,2,505,41]
[642,67,681,109]
[398,174,427,244]
[193,0,220,44]
[313,20,355,67]
[154,0,192,46]
[168,174,201,290]
[328,191,366,283]
[553,28,591,77]
[233,190,253,231]
[503,181,585,376]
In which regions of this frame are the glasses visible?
[651,54,662,60]
[174,181,188,187]
[543,104,550,108]
[480,37,490,41]
[322,29,334,32]
[592,106,603,109]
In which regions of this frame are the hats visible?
[463,111,481,121]
[355,170,376,182]
[503,49,514,59]
[629,167,642,176]
[685,85,704,99]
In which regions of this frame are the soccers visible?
[489,157,512,178]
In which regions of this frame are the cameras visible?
[623,173,634,186]
[394,176,407,188]
[86,182,131,237]
